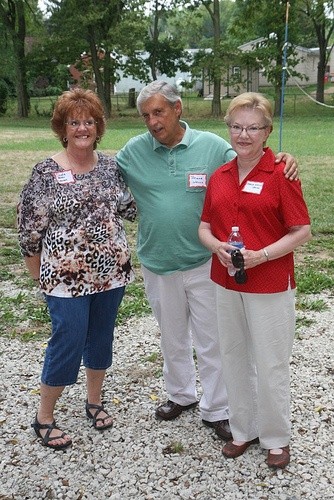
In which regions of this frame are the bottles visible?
[226,226,244,276]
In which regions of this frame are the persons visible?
[17,88,138,449]
[115,80,298,439]
[198,92,312,466]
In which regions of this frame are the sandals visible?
[85,399,113,430]
[30,413,72,448]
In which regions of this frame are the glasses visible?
[228,124,267,135]
[64,119,99,129]
[232,249,247,285]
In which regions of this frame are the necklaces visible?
[66,150,75,174]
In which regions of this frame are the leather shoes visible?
[202,419,232,439]
[268,444,290,467]
[156,400,198,419]
[222,437,259,457]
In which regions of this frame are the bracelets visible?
[262,247,270,262]
[33,278,40,281]
